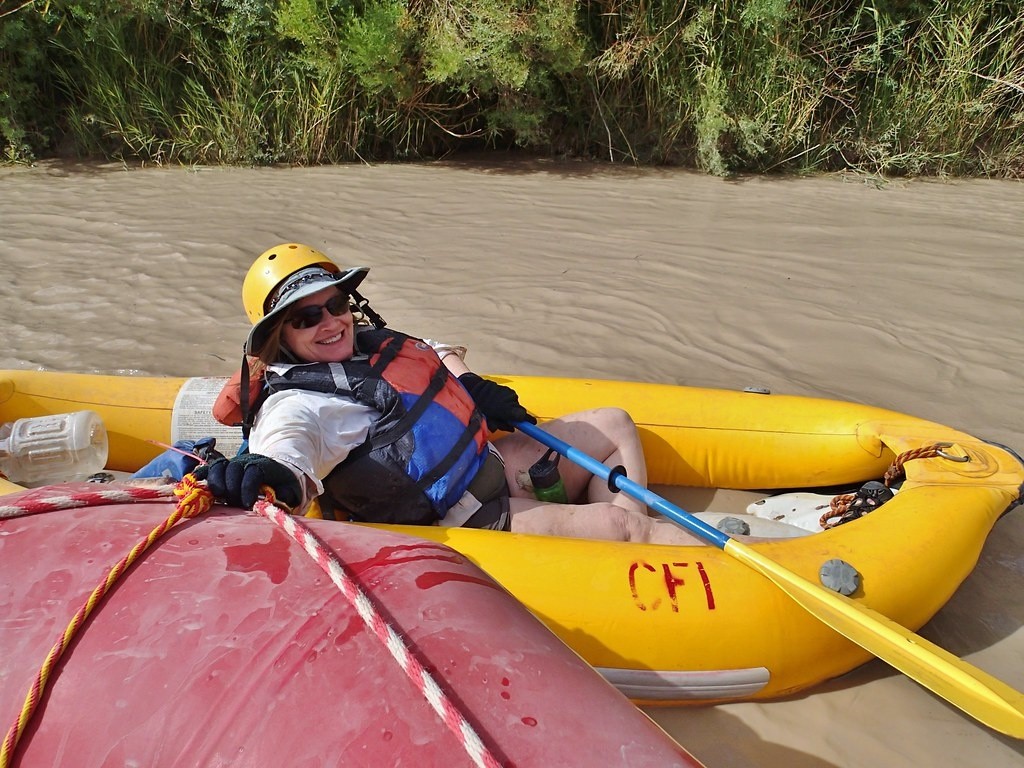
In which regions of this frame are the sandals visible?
[824,481,894,530]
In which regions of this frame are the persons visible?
[193,243,820,545]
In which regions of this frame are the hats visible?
[246,265,371,355]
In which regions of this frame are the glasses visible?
[282,295,348,329]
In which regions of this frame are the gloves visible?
[459,372,537,432]
[193,453,302,510]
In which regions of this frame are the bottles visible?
[528,448,568,505]
[0,410,108,489]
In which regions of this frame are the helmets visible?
[242,243,340,326]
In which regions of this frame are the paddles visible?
[508,413,1024,744]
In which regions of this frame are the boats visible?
[0,369,1024,767]
